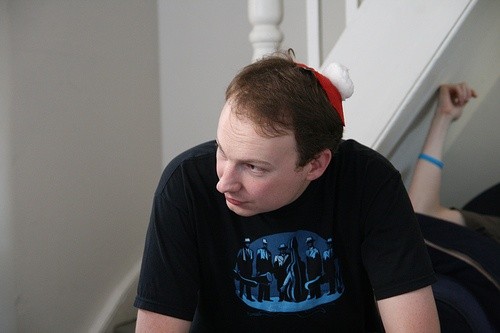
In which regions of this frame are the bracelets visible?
[419,153,444,169]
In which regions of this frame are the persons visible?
[133,51,442,333]
[409,82,500,244]
